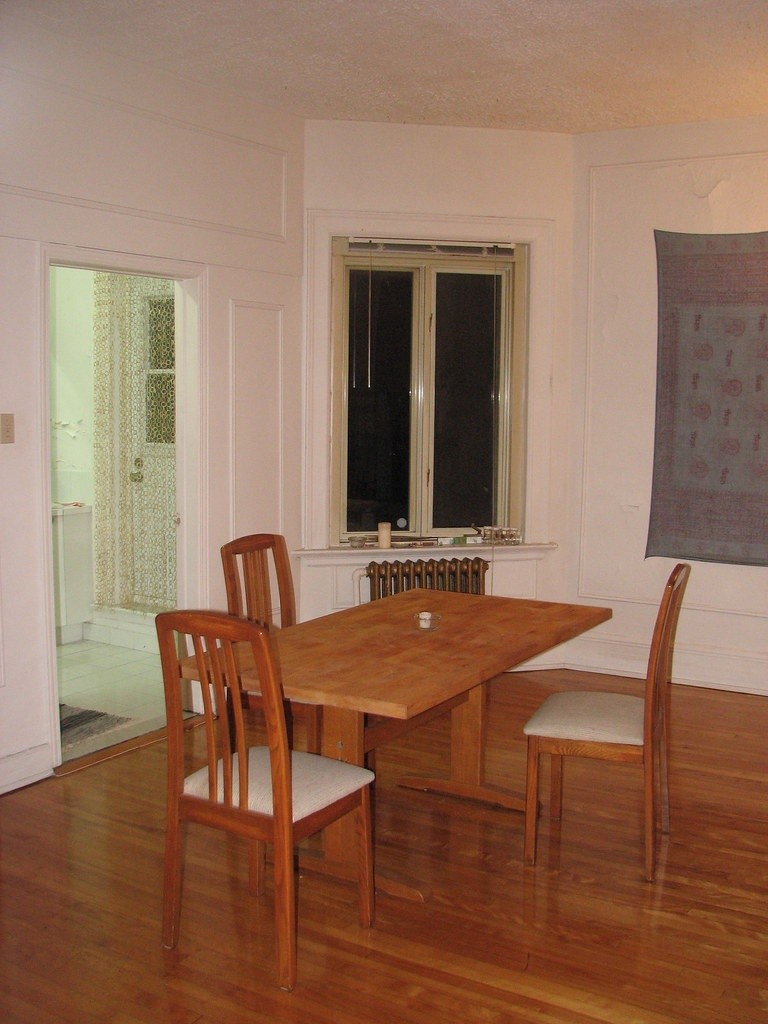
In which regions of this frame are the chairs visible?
[220,534,370,753]
[155,611,376,992]
[523,562,692,884]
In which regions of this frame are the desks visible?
[176,588,613,902]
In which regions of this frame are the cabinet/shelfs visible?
[52,505,93,646]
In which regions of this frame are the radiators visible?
[351,555,492,606]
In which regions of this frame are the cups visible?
[377,522,391,549]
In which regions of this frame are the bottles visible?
[483,526,522,545]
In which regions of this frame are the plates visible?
[391,541,412,547]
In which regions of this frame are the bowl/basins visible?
[414,612,441,631]
[348,537,366,549]
